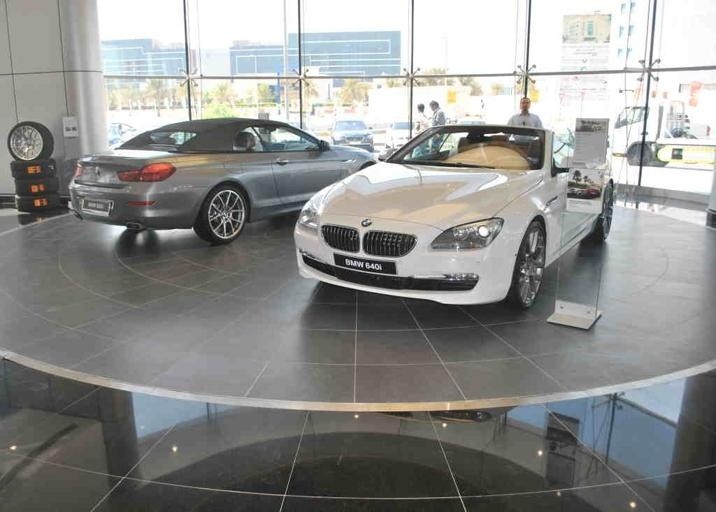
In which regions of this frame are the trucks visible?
[609,99,715,168]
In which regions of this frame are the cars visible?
[327,120,374,151]
[67,116,377,245]
[135,145,137,146]
[385,124,419,149]
[108,122,135,146]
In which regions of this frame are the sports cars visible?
[291,123,615,310]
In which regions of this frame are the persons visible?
[411,104,428,159]
[429,101,446,153]
[507,97,544,129]
